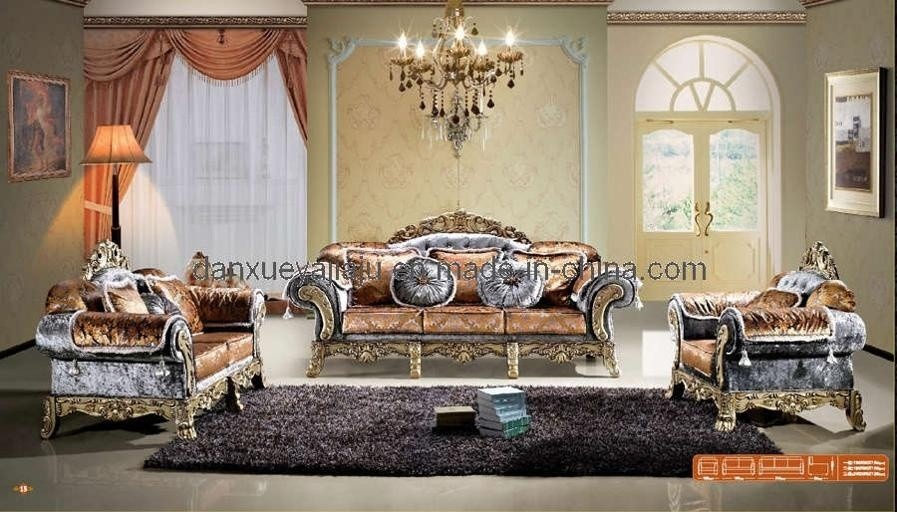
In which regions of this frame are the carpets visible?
[141,384,787,479]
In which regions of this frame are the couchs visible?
[34,237,268,445]
[281,206,644,380]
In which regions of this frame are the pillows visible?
[343,245,589,310]
[100,273,206,336]
[745,288,802,308]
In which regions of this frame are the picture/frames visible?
[4,68,73,186]
[822,65,887,220]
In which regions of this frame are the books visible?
[436,385,532,438]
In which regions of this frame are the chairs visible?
[663,239,868,433]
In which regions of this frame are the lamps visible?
[78,124,153,248]
[384,2,525,158]
